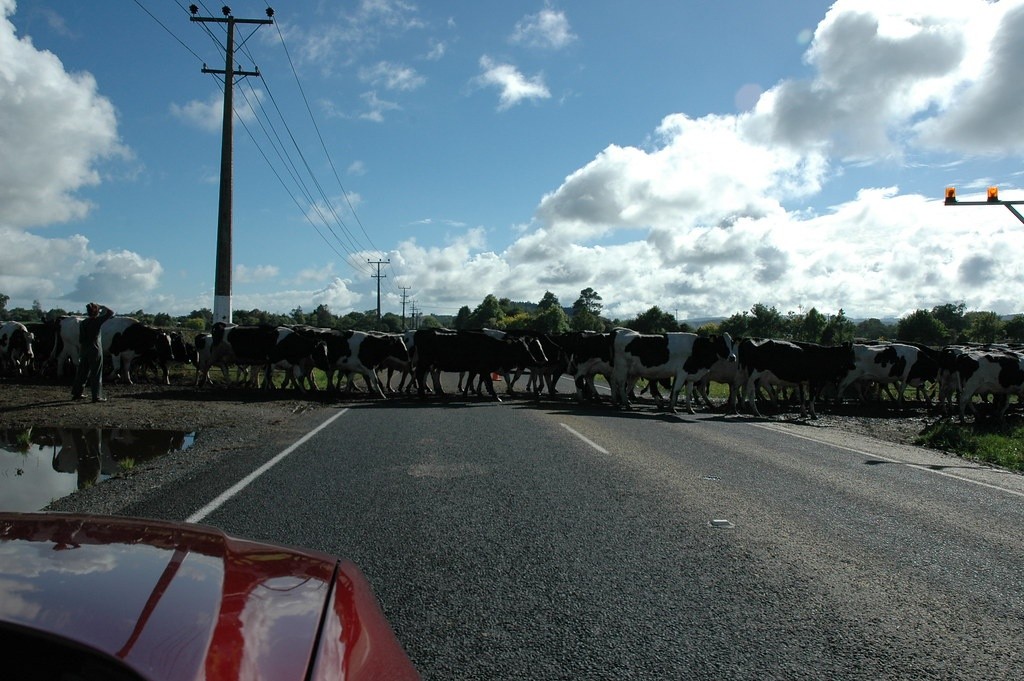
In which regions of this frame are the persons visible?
[71,303,113,402]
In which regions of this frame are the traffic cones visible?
[491,372,502,381]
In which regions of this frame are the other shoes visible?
[93,396,107,402]
[72,394,88,400]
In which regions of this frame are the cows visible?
[0,313,1024,421]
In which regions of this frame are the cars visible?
[1,513,428,681]
